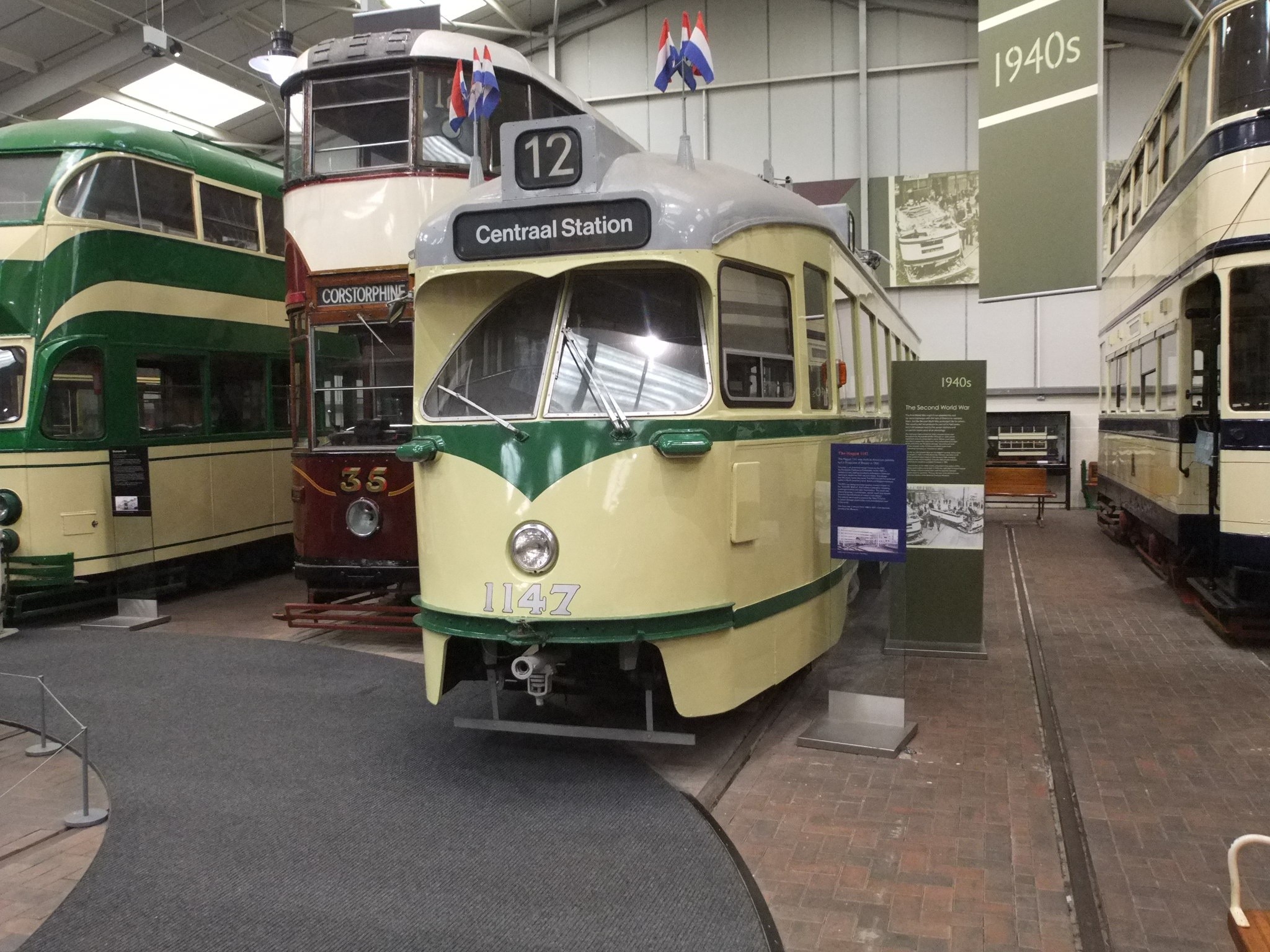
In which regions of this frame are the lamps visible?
[141,26,183,58]
[248,0,299,86]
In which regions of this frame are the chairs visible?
[1085,462,1099,486]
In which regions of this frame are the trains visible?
[394,12,925,746]
[0,118,372,619]
[272,28,649,633]
[1095,0,1270,646]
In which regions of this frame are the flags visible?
[468,47,485,122]
[654,16,679,93]
[448,59,469,133]
[676,10,697,92]
[479,45,500,119]
[684,10,715,84]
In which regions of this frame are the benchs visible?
[985,467,1058,528]
